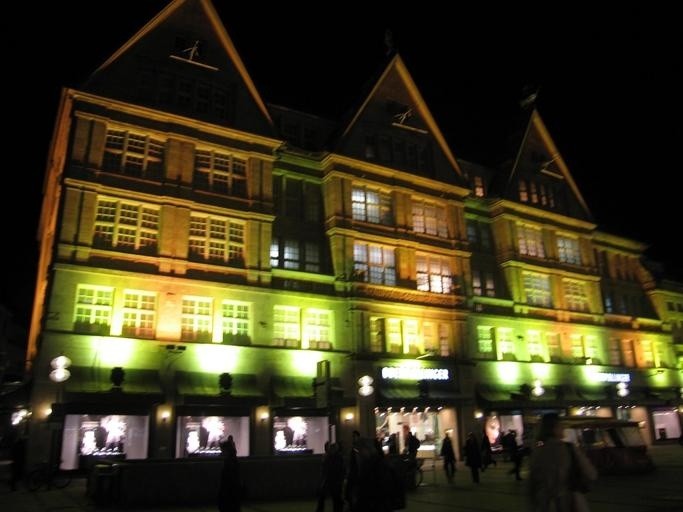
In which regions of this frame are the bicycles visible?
[25,458,71,492]
[398,455,422,490]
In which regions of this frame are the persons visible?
[463,425,537,483]
[7,428,36,492]
[531,411,599,511]
[217,433,238,458]
[216,446,242,512]
[312,428,422,511]
[438,432,456,483]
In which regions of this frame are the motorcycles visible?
[521,416,648,474]
